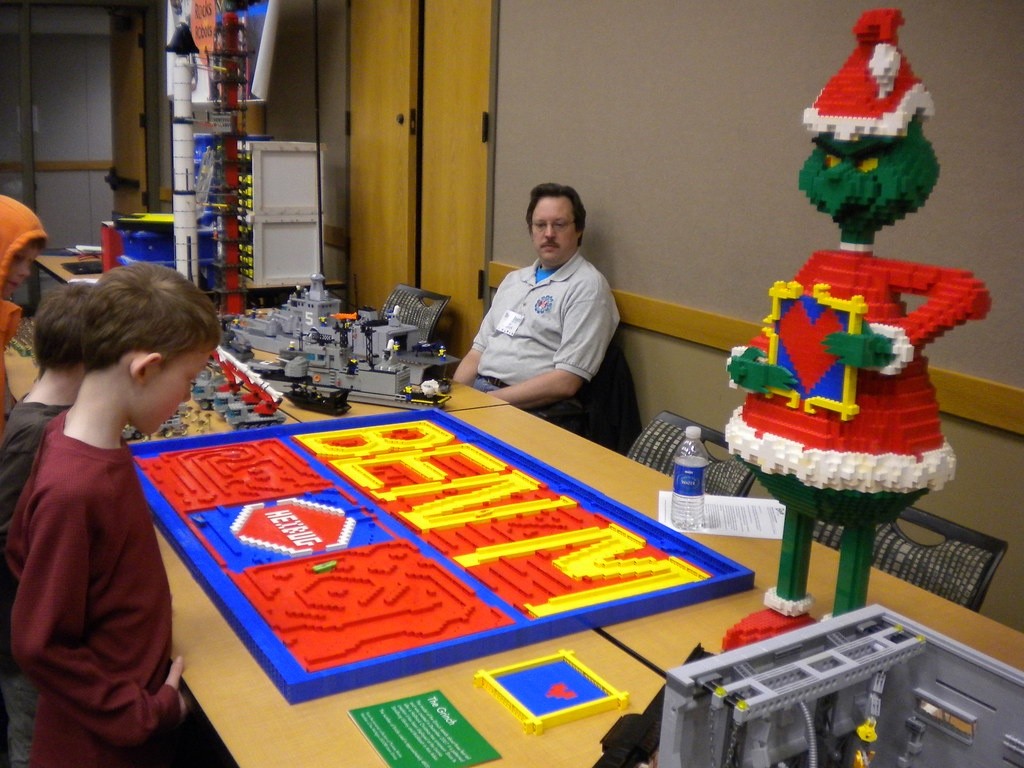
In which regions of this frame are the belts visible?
[480,375,510,388]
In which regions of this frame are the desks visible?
[4,256,1024,767]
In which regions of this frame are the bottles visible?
[670,425,711,531]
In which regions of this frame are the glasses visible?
[531,218,575,232]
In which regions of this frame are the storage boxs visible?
[99,134,276,292]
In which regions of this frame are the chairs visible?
[629,410,754,500]
[377,284,452,343]
[533,333,642,454]
[811,494,1009,612]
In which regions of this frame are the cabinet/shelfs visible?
[236,141,324,289]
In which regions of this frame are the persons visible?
[0,281,93,768]
[5,265,222,768]
[0,194,50,439]
[454,183,620,417]
